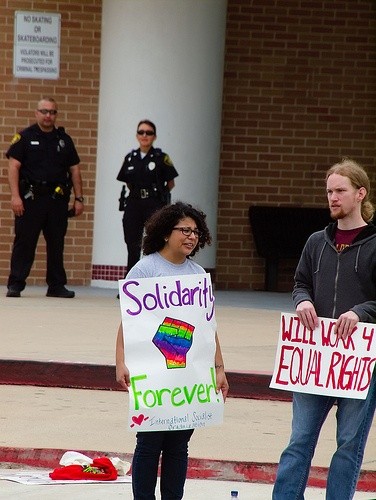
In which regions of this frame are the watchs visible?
[75,196,84,202]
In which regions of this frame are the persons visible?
[6,97,85,298]
[116,200,228,500]
[271,157,376,500]
[116,119,179,299]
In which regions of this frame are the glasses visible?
[173,227,202,238]
[38,109,57,115]
[137,130,155,136]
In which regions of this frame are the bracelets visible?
[215,365,224,369]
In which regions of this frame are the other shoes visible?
[7,288,20,297]
[46,286,75,298]
